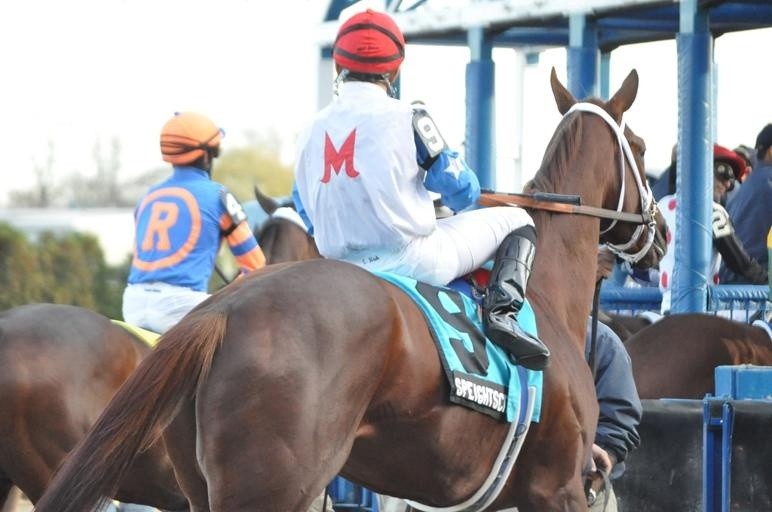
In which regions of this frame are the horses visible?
[622,312,772,400]
[0,183,325,512]
[33,65,670,512]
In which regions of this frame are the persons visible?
[580,120,771,315]
[290,7,552,372]
[119,112,268,336]
[581,317,644,512]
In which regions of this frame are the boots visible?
[484,235,550,371]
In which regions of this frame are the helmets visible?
[160,111,222,165]
[714,144,754,179]
[334,10,405,74]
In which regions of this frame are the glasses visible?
[717,163,735,193]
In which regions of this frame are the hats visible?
[755,125,772,152]
